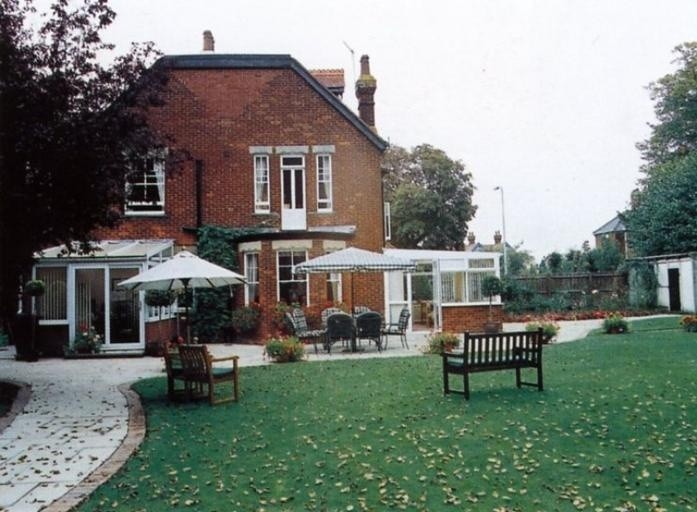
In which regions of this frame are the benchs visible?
[438,327,544,402]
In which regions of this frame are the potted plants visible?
[262,335,312,363]
[63,325,104,355]
[525,322,562,345]
[480,275,509,337]
[600,314,629,334]
[414,327,461,357]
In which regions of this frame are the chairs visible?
[284,305,412,356]
[163,343,240,406]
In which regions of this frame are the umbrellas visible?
[293,242,418,320]
[117,245,249,345]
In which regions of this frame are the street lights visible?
[168,146,203,252]
[493,186,507,275]
